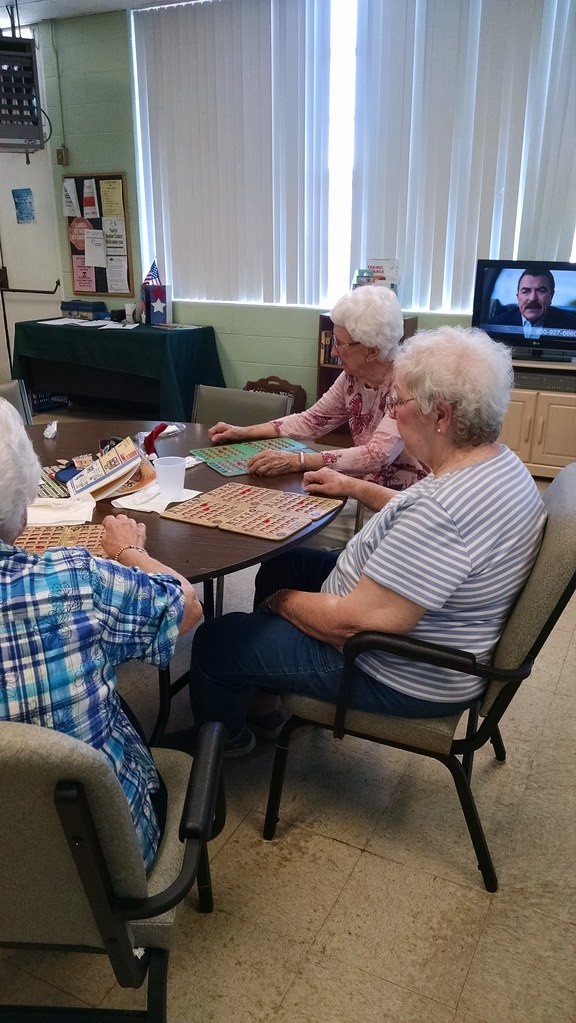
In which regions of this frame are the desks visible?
[12,317,226,423]
[21,420,350,752]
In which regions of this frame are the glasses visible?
[331,335,361,351]
[384,394,413,415]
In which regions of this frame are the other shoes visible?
[222,727,255,759]
[246,707,285,742]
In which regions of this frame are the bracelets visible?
[114,545,148,561]
[265,587,295,615]
[297,451,307,472]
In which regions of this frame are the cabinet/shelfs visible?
[315,312,418,448]
[495,357,576,478]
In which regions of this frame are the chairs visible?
[260,461,576,892]
[241,375,307,415]
[191,382,292,427]
[0,379,34,426]
[0,718,224,1023]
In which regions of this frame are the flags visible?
[143,261,162,285]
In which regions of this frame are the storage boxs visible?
[60,300,107,320]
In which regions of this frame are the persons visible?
[0,395,203,884]
[207,285,441,551]
[155,324,549,764]
[488,268,576,339]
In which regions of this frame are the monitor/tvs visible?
[471,259,575,362]
